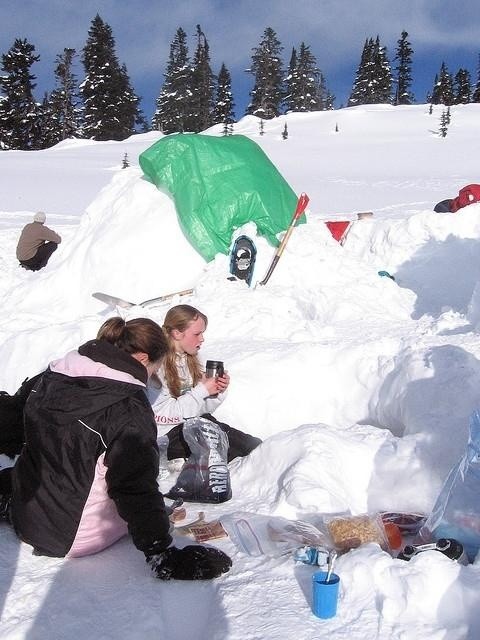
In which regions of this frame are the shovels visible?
[92,277,237,310]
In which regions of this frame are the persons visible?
[143,304,263,464]
[0,317,233,581]
[16,211,61,270]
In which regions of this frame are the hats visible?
[34,212,46,223]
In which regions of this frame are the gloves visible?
[147,545,232,580]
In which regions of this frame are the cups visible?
[311,571,340,619]
[204,359,224,398]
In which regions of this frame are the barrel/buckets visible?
[310,550,341,621]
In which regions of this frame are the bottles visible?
[397,540,469,571]
